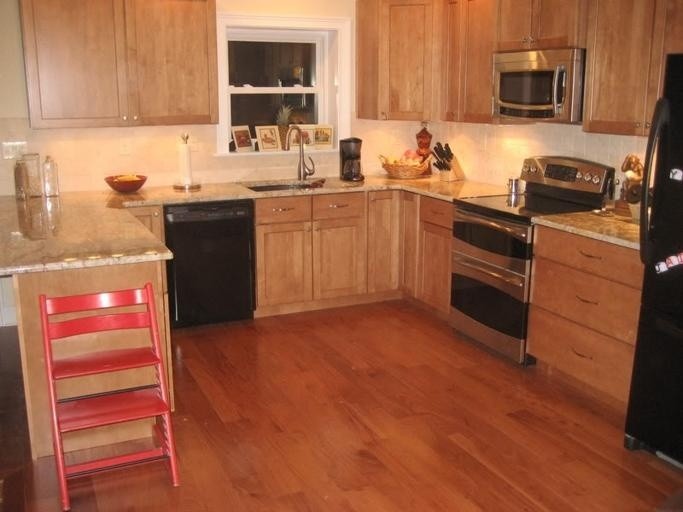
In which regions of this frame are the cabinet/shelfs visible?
[398,188,420,307]
[249,189,368,321]
[17,0,221,132]
[368,185,404,303]
[523,221,646,408]
[122,201,166,247]
[414,192,454,318]
[352,0,682,142]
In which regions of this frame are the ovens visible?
[446,206,534,366]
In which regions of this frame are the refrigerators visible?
[618,52,682,475]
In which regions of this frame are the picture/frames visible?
[287,123,316,151]
[230,123,254,153]
[310,123,334,150]
[253,125,282,153]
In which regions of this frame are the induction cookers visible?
[452,192,600,225]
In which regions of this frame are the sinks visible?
[243,178,317,193]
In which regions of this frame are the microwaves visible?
[489,48,582,125]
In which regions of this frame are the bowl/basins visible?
[105,173,147,193]
[104,192,146,209]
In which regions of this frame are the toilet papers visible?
[177,142,192,186]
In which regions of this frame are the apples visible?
[388,148,423,165]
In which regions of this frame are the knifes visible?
[431,142,453,169]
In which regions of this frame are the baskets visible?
[377,153,430,179]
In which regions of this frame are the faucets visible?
[284,124,316,180]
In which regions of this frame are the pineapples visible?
[276,104,293,150]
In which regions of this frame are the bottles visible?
[43,157,59,196]
[14,160,31,201]
[21,152,42,198]
[16,197,61,240]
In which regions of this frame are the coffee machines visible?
[339,137,364,181]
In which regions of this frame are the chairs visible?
[37,282,181,512]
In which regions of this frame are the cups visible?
[627,202,640,224]
[508,178,519,193]
[506,193,519,207]
[605,195,615,212]
